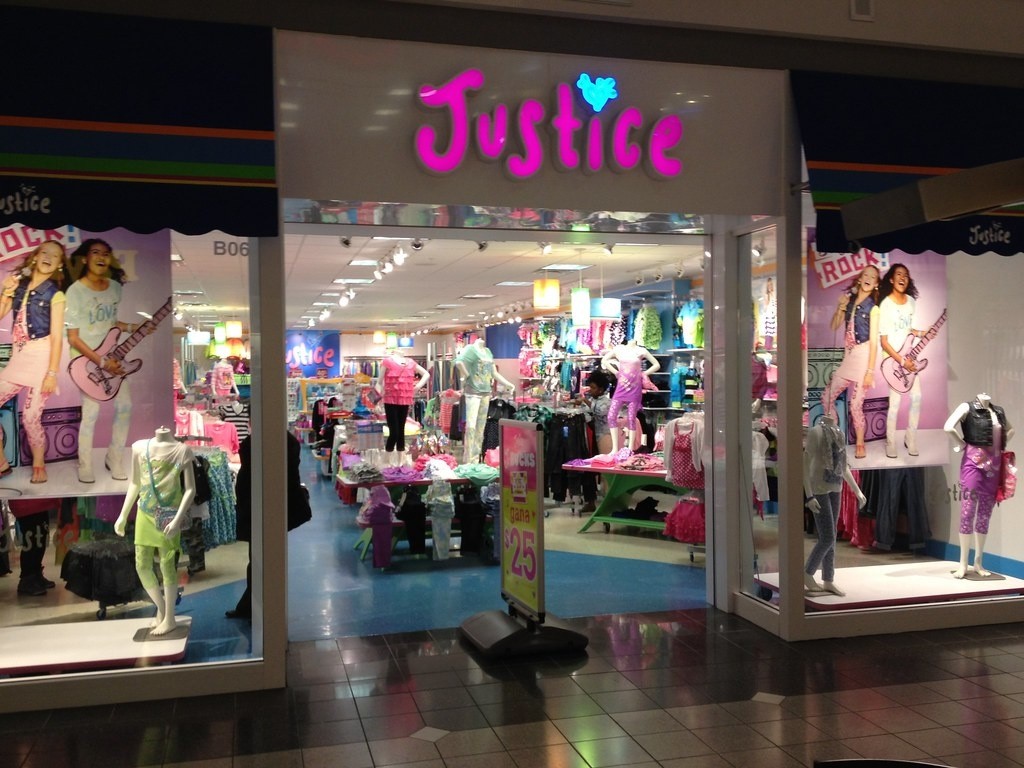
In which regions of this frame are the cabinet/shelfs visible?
[641,354,685,411]
[297,379,342,421]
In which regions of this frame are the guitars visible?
[68,297,173,403]
[882,309,951,396]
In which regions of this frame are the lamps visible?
[373,266,383,280]
[427,324,438,332]
[380,256,393,275]
[603,242,616,255]
[752,237,766,258]
[536,242,552,255]
[373,318,414,350]
[339,293,349,308]
[340,237,352,249]
[700,259,704,271]
[479,300,534,328]
[308,306,332,327]
[411,238,424,251]
[636,273,644,285]
[393,247,408,266]
[474,241,489,252]
[571,247,591,330]
[534,255,561,310]
[675,260,686,278]
[655,268,664,282]
[174,308,243,346]
[345,288,356,300]
[590,255,623,320]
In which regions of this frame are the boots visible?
[885,408,898,458]
[904,402,921,456]
[76,420,95,482]
[104,413,130,479]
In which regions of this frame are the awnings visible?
[789,70,1024,258]
[1,0,284,240]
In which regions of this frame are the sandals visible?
[0,460,13,478]
[30,465,47,484]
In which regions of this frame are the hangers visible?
[178,407,224,424]
[689,289,703,301]
[552,402,584,418]
[681,402,705,422]
[72,519,135,545]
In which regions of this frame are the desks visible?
[560,465,689,534]
[313,450,333,477]
[334,473,500,572]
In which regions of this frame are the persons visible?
[226,402,312,630]
[455,338,516,464]
[0,237,157,484]
[373,350,431,467]
[577,371,617,513]
[112,353,240,635]
[8,498,61,597]
[820,263,938,459]
[602,338,1015,597]
[762,277,777,350]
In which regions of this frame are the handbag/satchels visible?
[155,506,192,530]
[995,451,1017,507]
[366,388,382,405]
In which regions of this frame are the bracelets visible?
[1,290,14,297]
[806,496,814,503]
[917,330,922,338]
[866,368,874,373]
[901,357,906,366]
[127,322,133,334]
[99,357,106,368]
[838,306,847,312]
[47,369,57,376]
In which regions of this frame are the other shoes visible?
[855,445,866,459]
[225,610,247,618]
[579,504,596,512]
[37,575,55,589]
[17,578,47,596]
